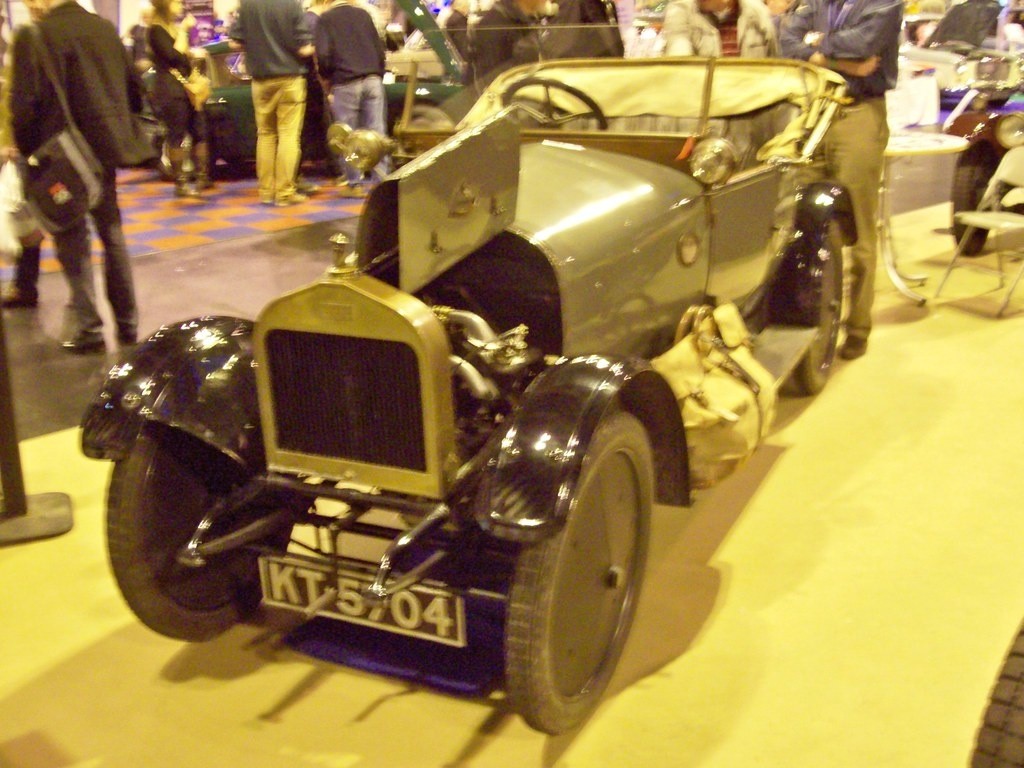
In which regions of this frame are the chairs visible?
[931,144,1024,322]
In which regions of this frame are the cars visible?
[952,78,1024,257]
[201,0,472,171]
[76,52,858,746]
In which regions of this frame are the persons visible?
[129,0,897,362]
[0,0,141,356]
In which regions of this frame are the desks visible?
[863,130,975,311]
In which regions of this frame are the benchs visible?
[462,51,829,161]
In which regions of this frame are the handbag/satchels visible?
[184,74,212,112]
[18,128,105,236]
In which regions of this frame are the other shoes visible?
[118,329,138,346]
[174,187,201,199]
[296,175,320,194]
[259,189,274,203]
[274,194,310,207]
[0,280,39,308]
[841,335,867,361]
[60,339,107,355]
[194,174,215,191]
